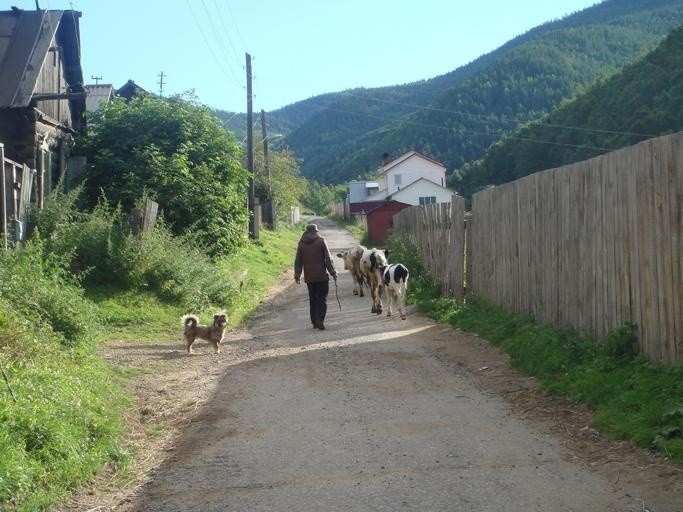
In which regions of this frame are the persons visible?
[295,223,338,330]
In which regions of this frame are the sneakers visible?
[313,320,324,330]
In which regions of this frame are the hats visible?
[306,224,319,232]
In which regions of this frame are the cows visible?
[379,262,409,320]
[359,248,391,315]
[336,245,367,297]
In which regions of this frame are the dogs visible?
[179,313,229,355]
